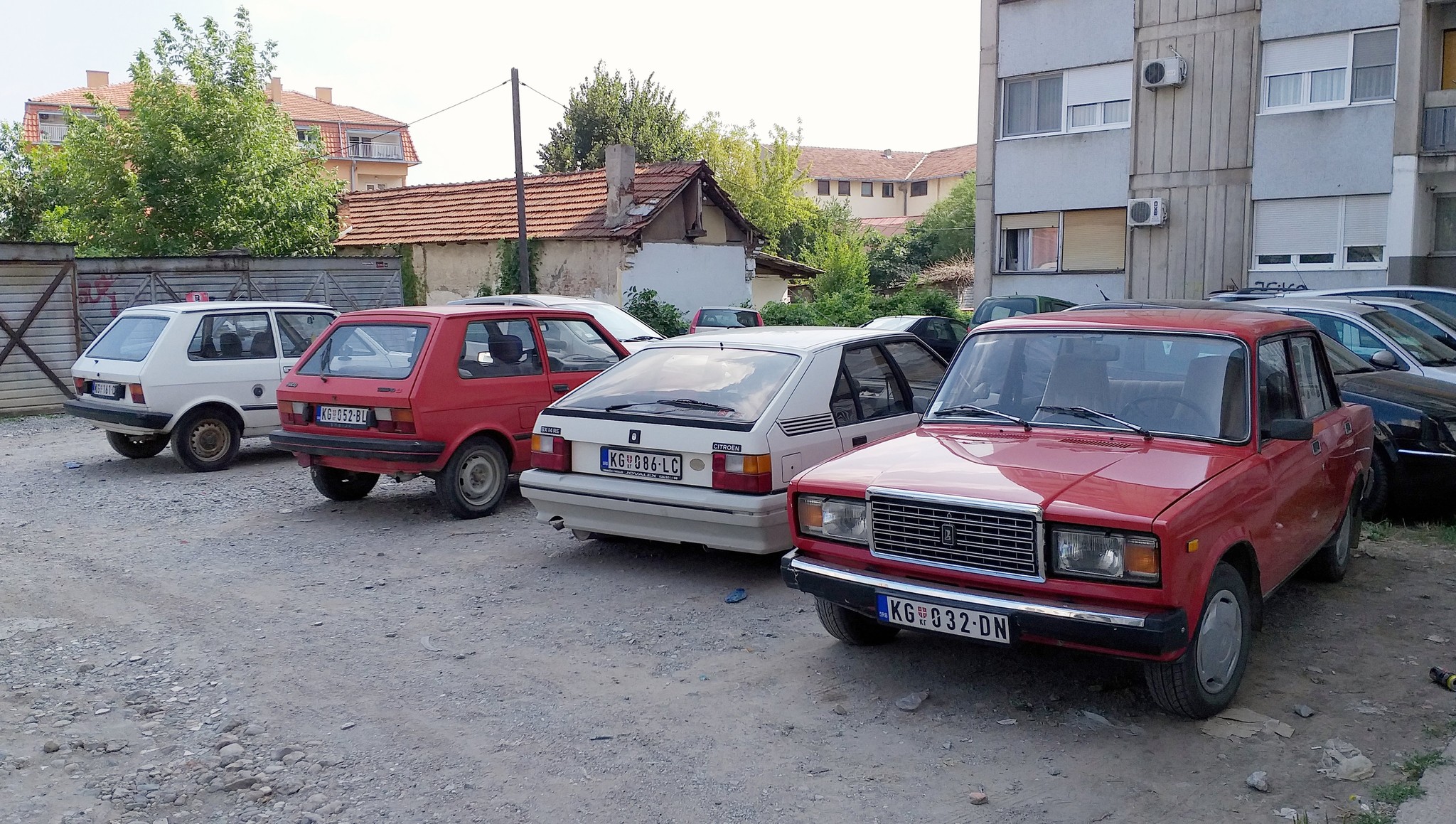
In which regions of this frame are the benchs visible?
[1106,379,1291,429]
[338,365,471,379]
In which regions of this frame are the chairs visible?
[219,333,245,359]
[1032,353,1109,427]
[1175,356,1246,438]
[832,376,875,425]
[459,340,487,376]
[488,334,530,375]
[250,331,274,357]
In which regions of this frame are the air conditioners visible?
[1140,55,1184,88]
[1126,197,1166,228]
[40,114,55,122]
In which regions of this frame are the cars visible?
[863,283,1455,521]
[454,293,668,369]
[62,299,413,472]
[268,305,633,517]
[687,305,767,335]
[519,326,998,557]
[778,307,1377,718]
